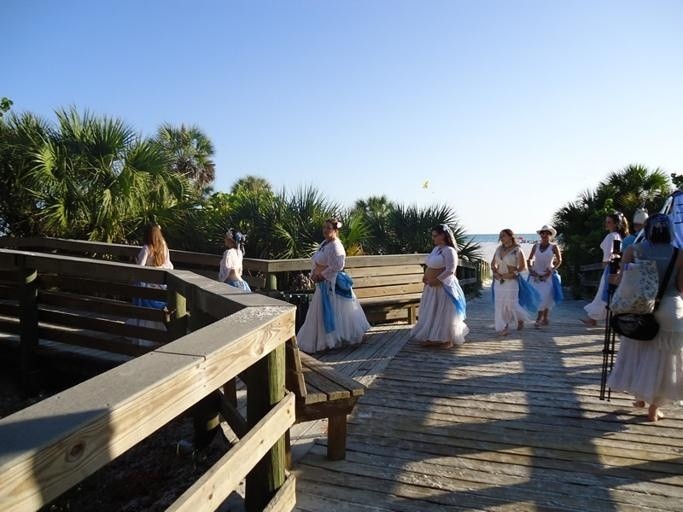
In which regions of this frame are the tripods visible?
[600,291,616,402]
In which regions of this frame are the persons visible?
[409,224,470,350]
[579,211,630,327]
[622,208,649,252]
[219,228,252,292]
[526,225,565,326]
[491,229,544,336]
[607,213,683,422]
[123,224,174,347]
[296,218,371,354]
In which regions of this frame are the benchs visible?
[223,336,365,470]
[343,265,426,326]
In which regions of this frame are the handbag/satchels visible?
[610,260,659,340]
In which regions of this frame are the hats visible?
[537,226,557,238]
